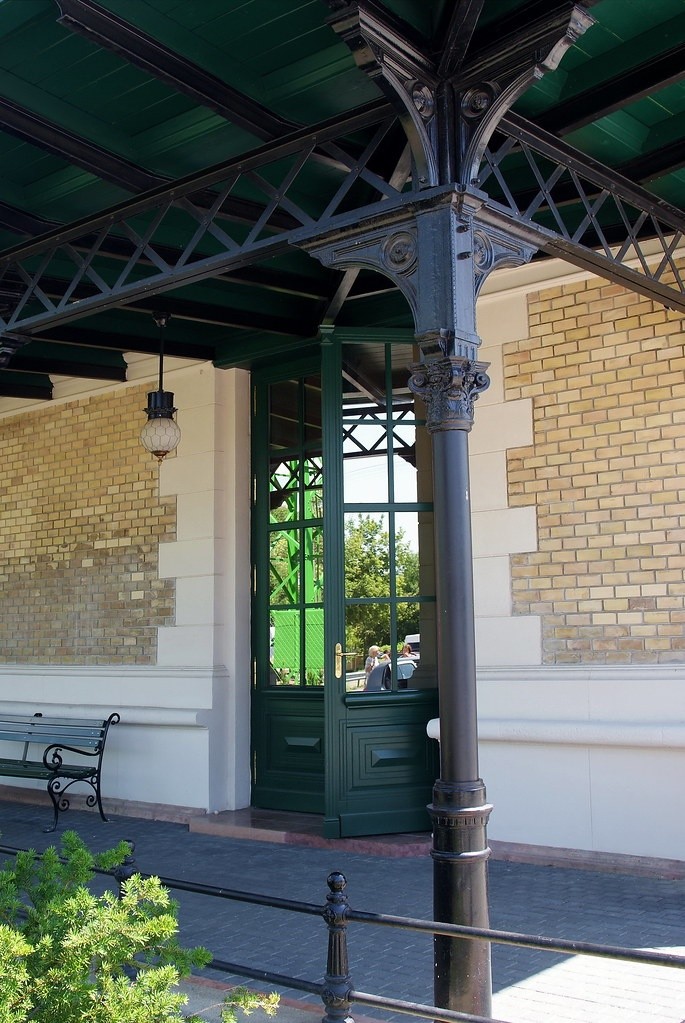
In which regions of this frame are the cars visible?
[363,652,420,691]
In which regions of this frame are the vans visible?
[403,634,420,665]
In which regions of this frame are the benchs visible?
[0,712,120,833]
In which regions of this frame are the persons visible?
[364,645,379,688]
[399,644,414,657]
[381,650,390,662]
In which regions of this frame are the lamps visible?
[138,294,182,466]
